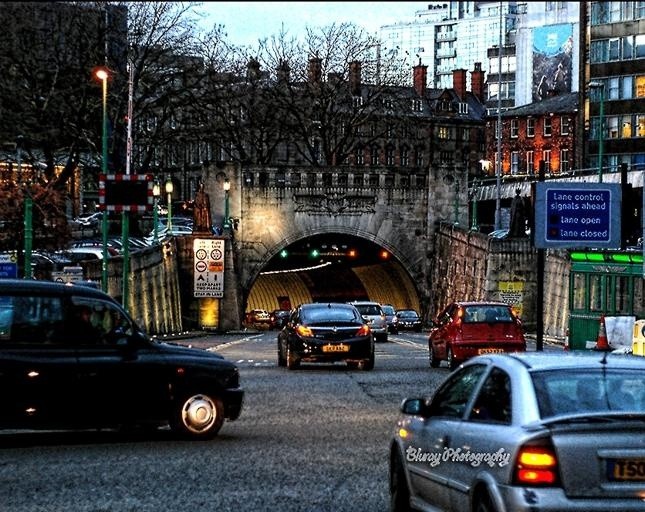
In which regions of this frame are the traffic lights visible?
[281,250,288,258]
[311,249,319,257]
[381,250,389,258]
[349,250,356,257]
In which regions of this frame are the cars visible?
[396,309,424,334]
[0,275,246,445]
[346,300,388,342]
[276,301,376,371]
[246,309,290,329]
[387,347,645,511]
[427,300,529,366]
[0,209,193,281]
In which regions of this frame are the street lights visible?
[163,181,175,233]
[150,184,162,245]
[94,67,110,294]
[589,81,605,185]
[222,178,232,229]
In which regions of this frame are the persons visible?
[575,377,606,410]
[553,62,569,95]
[75,300,115,350]
[536,74,550,101]
[192,183,212,232]
[563,36,573,59]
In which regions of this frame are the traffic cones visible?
[593,313,614,350]
[563,328,570,350]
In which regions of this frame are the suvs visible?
[381,303,398,333]
